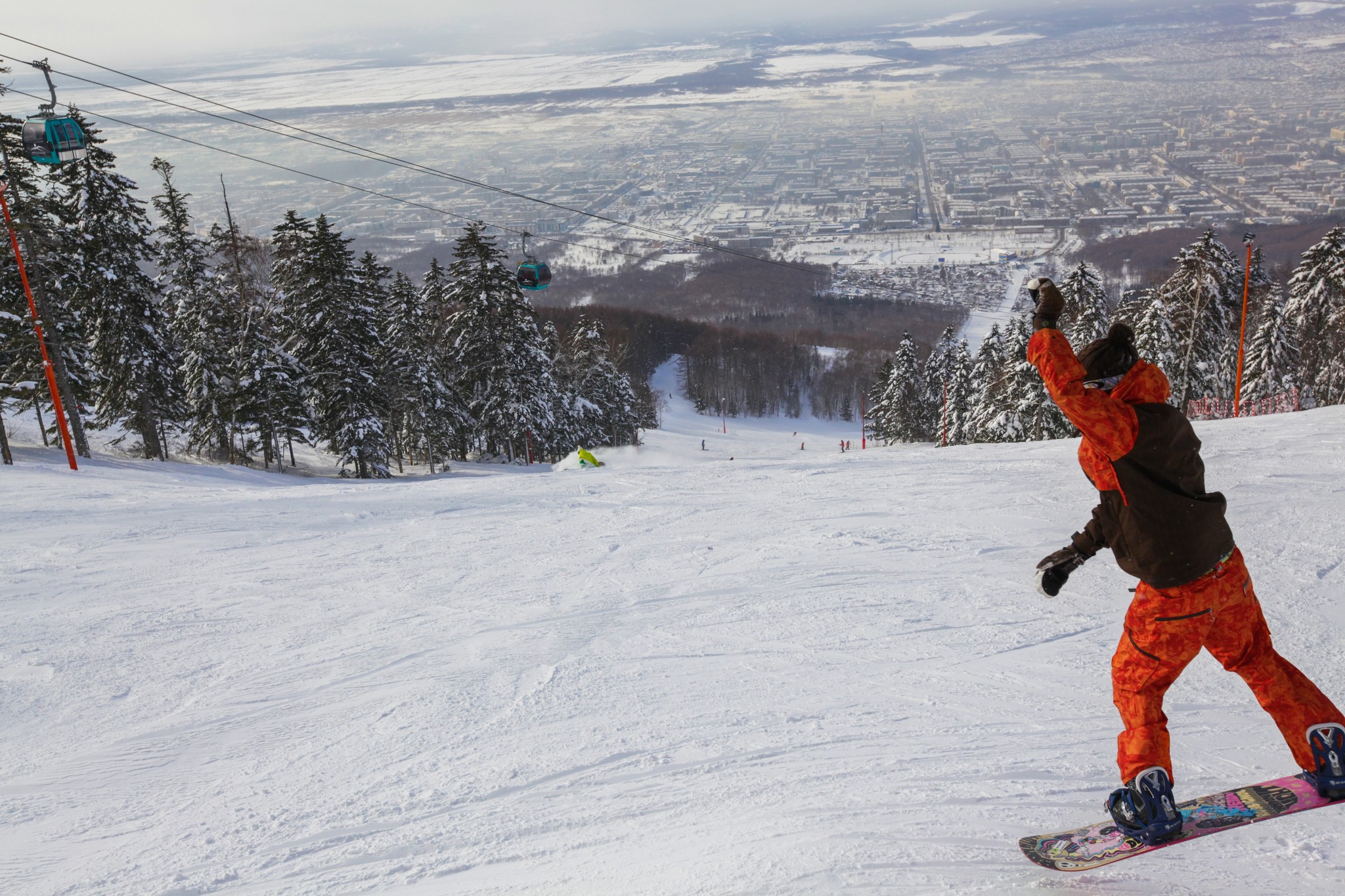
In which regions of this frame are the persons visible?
[1025,275,1345,847]
[700,432,851,453]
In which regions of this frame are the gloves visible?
[1037,545,1088,597]
[1028,276,1065,335]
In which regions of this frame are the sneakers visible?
[1106,765,1184,846]
[1301,723,1345,799]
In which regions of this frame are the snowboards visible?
[1017,769,1345,873]
[799,448,806,451]
[563,462,606,472]
[845,448,851,450]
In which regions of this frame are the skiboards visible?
[837,450,850,454]
[702,449,709,451]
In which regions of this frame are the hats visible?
[1073,324,1141,391]
